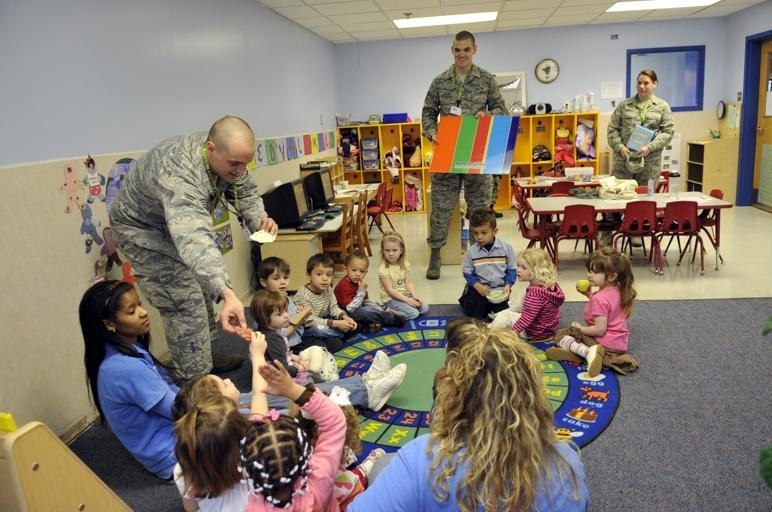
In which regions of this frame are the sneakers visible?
[360,363,407,414]
[390,313,408,330]
[210,352,245,374]
[357,447,388,479]
[631,236,643,248]
[491,207,504,219]
[358,318,384,334]
[359,349,392,384]
[598,231,614,250]
[545,346,582,362]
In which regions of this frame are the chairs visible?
[366,186,395,235]
[321,198,357,274]
[511,164,734,275]
[337,189,372,259]
[367,181,389,227]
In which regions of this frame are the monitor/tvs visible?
[260,179,310,229]
[304,168,335,210]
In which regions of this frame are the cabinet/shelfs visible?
[334,111,601,216]
[426,177,468,265]
[683,134,740,206]
[300,162,344,188]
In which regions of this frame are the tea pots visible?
[507,100,529,116]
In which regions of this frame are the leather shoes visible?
[583,343,606,379]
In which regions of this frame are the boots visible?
[426,247,442,280]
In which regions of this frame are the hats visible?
[625,151,645,174]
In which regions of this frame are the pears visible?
[577,279,591,293]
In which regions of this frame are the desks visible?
[248,182,381,296]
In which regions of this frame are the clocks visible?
[535,58,560,83]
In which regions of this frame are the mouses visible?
[325,215,335,219]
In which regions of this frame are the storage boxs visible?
[342,137,381,171]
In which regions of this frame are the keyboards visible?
[324,206,340,212]
[296,219,324,231]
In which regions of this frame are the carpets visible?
[68,295,771,512]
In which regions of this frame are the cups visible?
[332,180,349,195]
[575,279,592,293]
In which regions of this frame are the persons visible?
[378,234,429,320]
[256,257,327,354]
[291,253,358,340]
[109,116,278,388]
[79,280,407,481]
[546,247,636,376]
[347,329,589,510]
[486,247,565,340]
[459,208,517,323]
[174,374,385,510]
[238,359,346,511]
[422,31,504,279]
[250,289,303,378]
[599,69,674,247]
[490,175,503,218]
[333,251,395,329]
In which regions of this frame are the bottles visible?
[647,178,655,198]
[668,170,683,199]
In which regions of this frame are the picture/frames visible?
[489,71,526,112]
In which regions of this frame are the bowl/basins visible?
[485,286,509,304]
